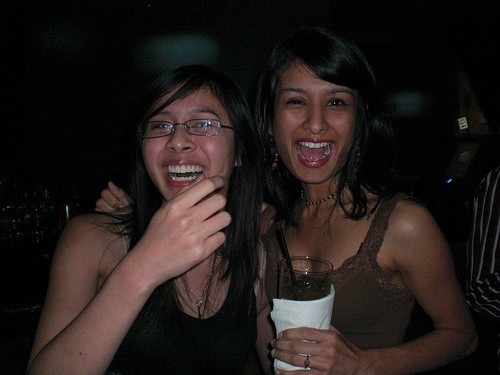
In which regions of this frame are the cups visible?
[428,93,494,203]
[271,256,335,372]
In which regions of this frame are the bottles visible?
[0,178,81,257]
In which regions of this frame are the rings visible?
[304,356,309,368]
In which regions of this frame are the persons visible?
[386,63,500,375]
[27,64,275,375]
[97,25,478,375]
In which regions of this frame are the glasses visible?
[137,114,236,140]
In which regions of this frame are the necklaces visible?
[181,250,219,316]
[300,185,347,205]
